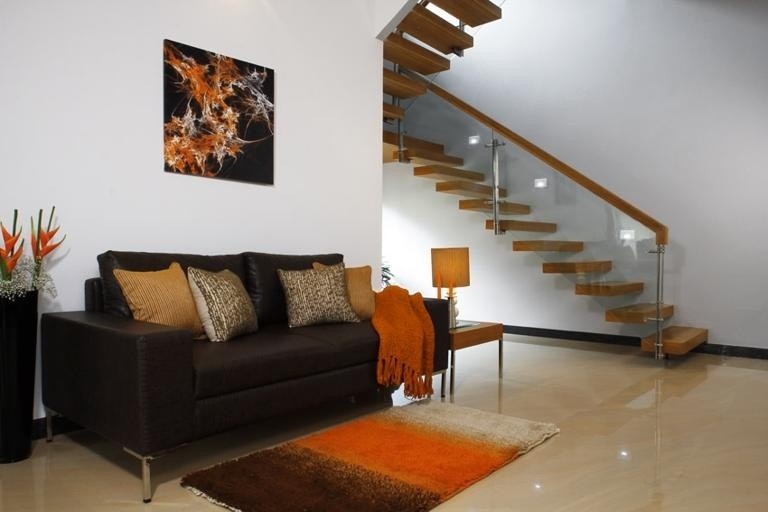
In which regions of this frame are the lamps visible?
[431,248,471,329]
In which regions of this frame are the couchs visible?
[39,250,450,502]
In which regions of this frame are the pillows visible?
[311,262,376,322]
[276,264,361,329]
[187,267,258,343]
[113,263,208,339]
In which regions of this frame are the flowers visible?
[0,204,67,298]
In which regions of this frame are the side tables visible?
[449,319,504,394]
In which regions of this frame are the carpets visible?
[180,399,560,512]
